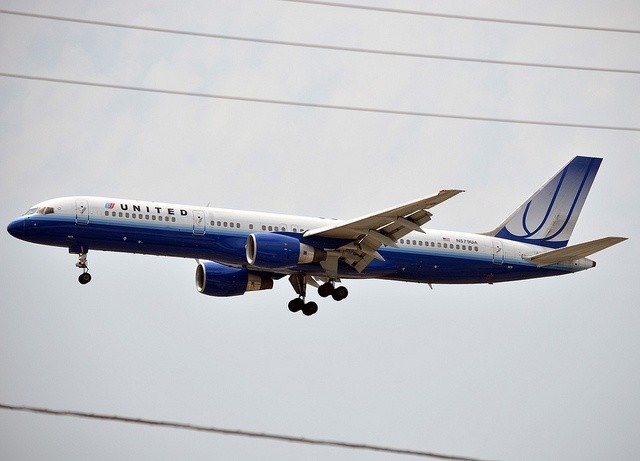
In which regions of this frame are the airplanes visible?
[7,156,629,315]
